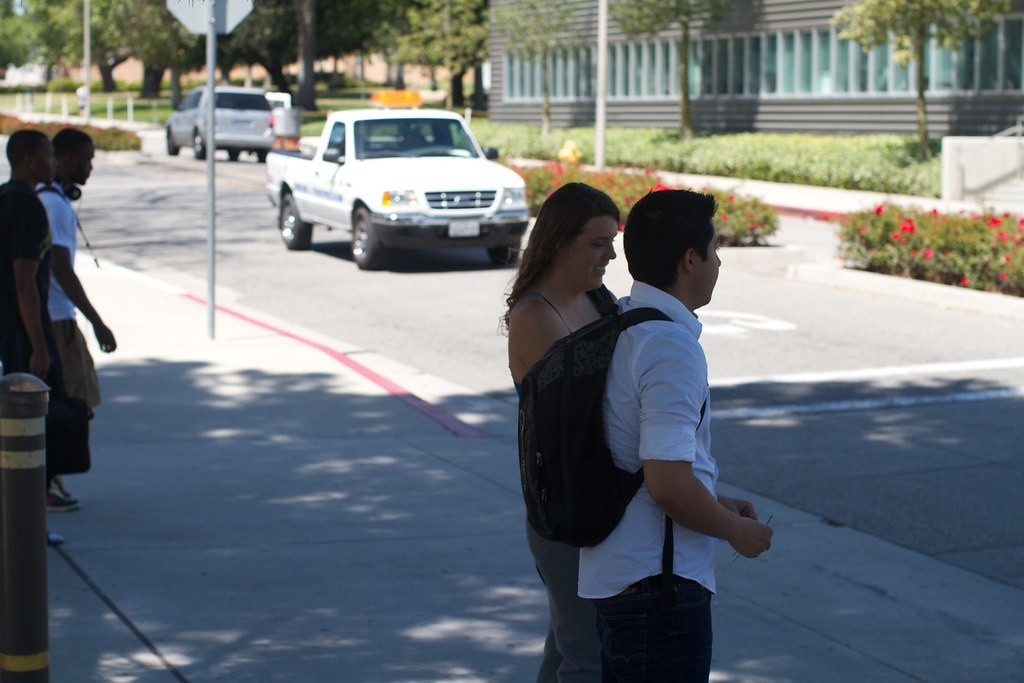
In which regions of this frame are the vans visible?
[163,84,277,162]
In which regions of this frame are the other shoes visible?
[51,478,71,498]
[46,488,80,511]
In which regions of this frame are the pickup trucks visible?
[264,86,532,272]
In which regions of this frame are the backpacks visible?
[519,304,706,547]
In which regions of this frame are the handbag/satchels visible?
[45,398,94,475]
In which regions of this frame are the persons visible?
[576,189,774,683]
[0,127,118,547]
[403,129,426,150]
[499,182,621,683]
